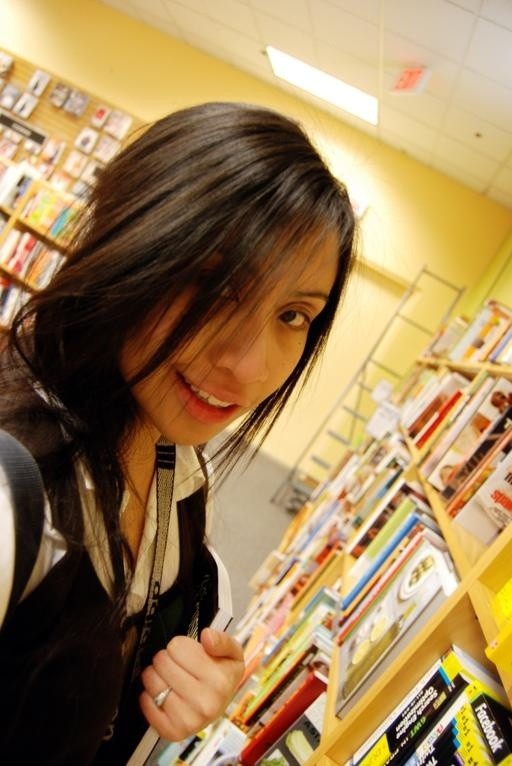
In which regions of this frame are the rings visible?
[151,688,173,710]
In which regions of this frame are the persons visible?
[1,100,357,766]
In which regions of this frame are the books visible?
[128,298,512,766]
[1,46,133,334]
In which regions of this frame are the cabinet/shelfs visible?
[0,45,150,341]
[144,330,511,765]
